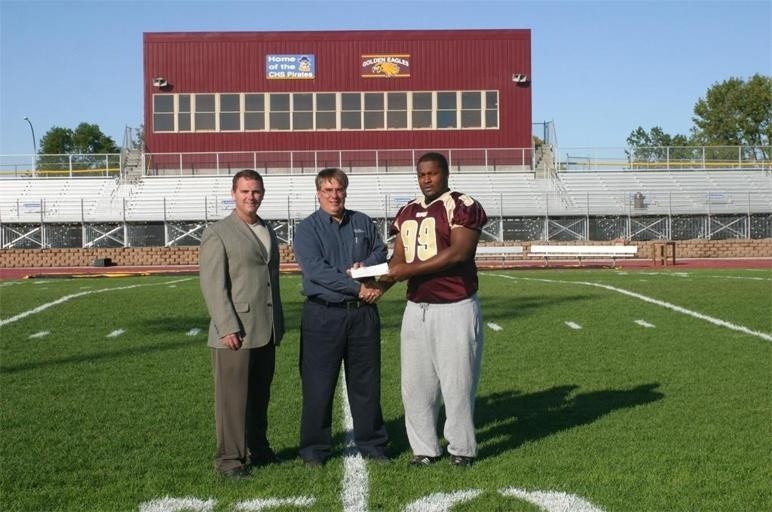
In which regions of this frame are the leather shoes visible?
[304,454,394,472]
[222,456,291,480]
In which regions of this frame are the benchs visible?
[529,245,638,261]
[475,246,524,260]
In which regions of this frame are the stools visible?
[653,242,676,268]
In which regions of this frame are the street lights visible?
[23,115,41,179]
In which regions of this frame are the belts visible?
[309,295,365,309]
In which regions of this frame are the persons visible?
[289,168,388,468]
[198,170,285,483]
[359,153,488,470]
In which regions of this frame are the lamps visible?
[153,77,169,90]
[512,73,528,86]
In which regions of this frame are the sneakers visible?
[412,454,441,469]
[452,455,473,470]
